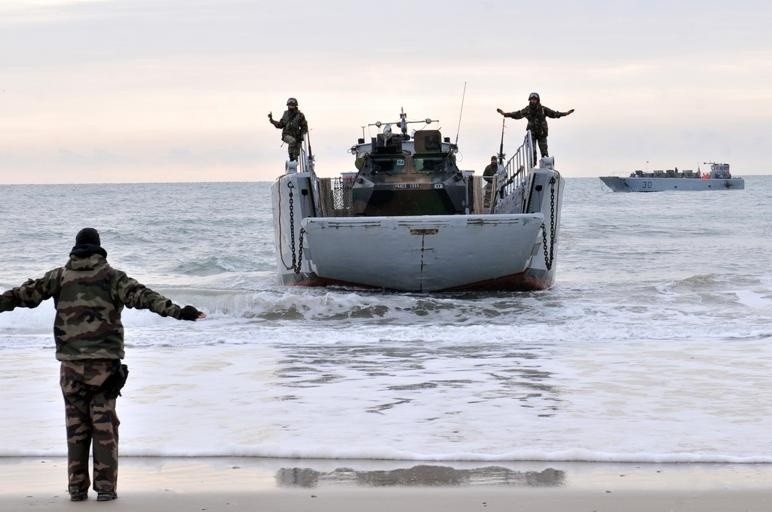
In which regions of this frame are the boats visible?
[599,162,745,193]
[263,82,567,297]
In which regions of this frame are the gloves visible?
[178,305,203,321]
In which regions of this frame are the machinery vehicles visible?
[344,128,469,217]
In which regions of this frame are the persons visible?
[497,92,574,165]
[0,227,207,503]
[482,156,506,199]
[266,96,309,162]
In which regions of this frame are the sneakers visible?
[71,493,88,502]
[97,492,118,501]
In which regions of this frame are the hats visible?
[76,228,101,245]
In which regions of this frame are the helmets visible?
[528,93,539,100]
[287,98,298,106]
